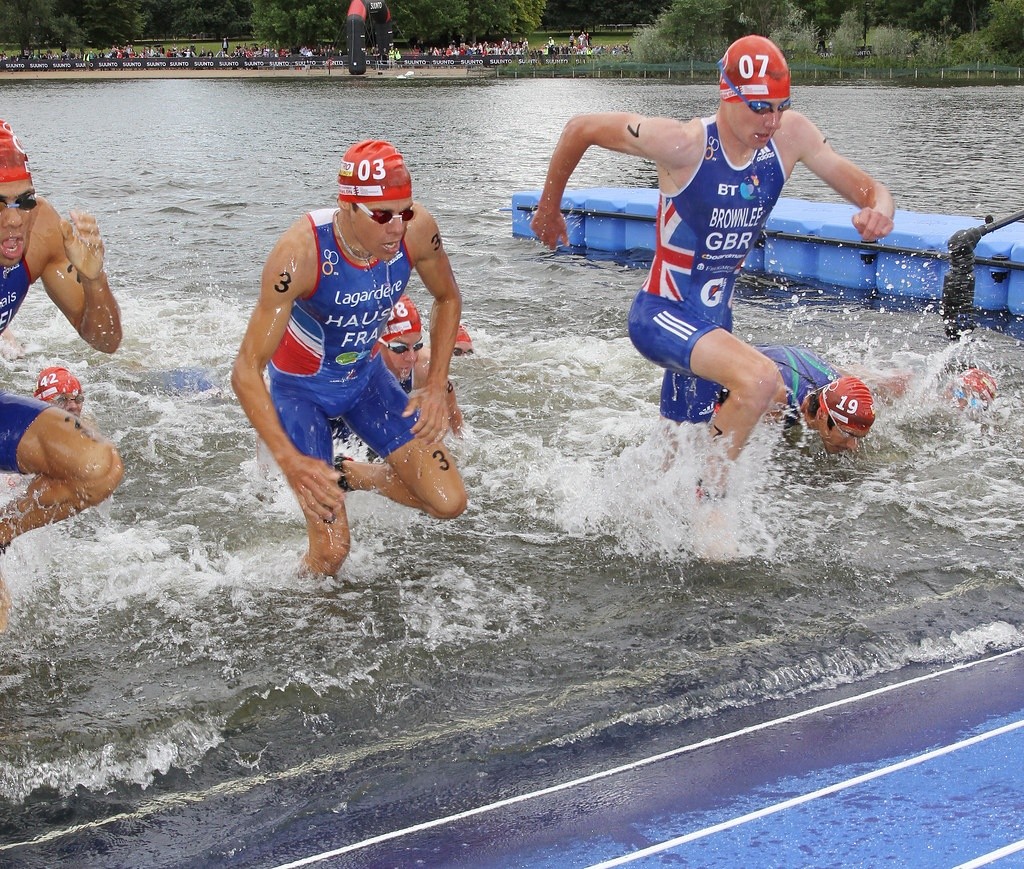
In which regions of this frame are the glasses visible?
[52,394,84,404]
[746,97,793,114]
[385,339,424,353]
[0,194,37,212]
[367,207,415,225]
[453,347,474,357]
[839,428,865,441]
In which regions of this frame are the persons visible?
[945,368,997,410]
[378,292,464,436]
[532,31,633,59]
[0,44,215,60]
[453,326,472,355]
[33,366,83,418]
[231,139,467,579]
[222,37,228,53]
[531,34,896,497]
[405,34,529,67]
[216,43,404,70]
[0,119,124,632]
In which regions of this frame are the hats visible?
[718,35,791,102]
[33,366,83,401]
[338,139,412,203]
[455,326,471,343]
[376,295,422,344]
[819,377,877,432]
[0,119,33,182]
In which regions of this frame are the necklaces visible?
[334,211,374,261]
